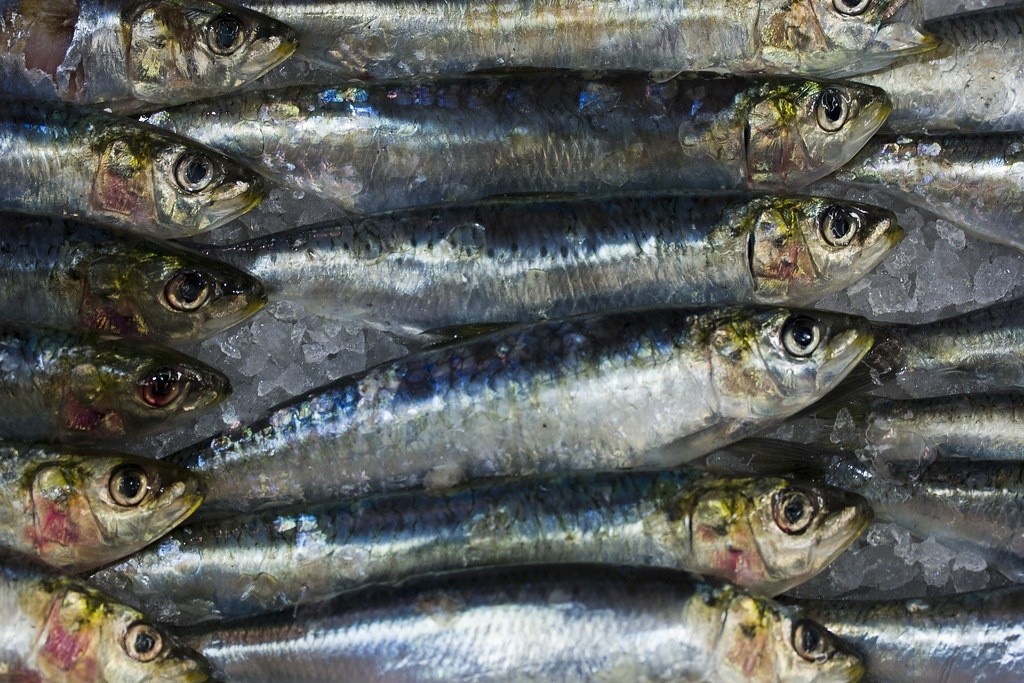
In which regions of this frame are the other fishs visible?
[1,0,1024,682]
